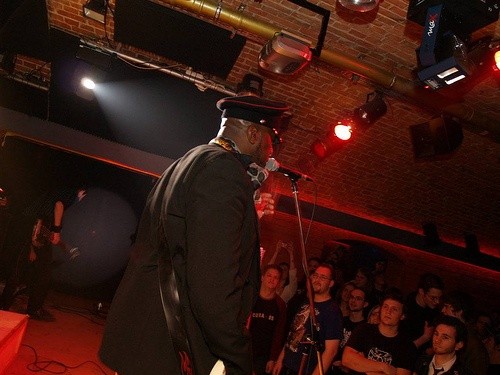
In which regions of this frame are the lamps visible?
[333,97,388,141]
[83,0,107,25]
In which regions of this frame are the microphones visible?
[266,158,315,183]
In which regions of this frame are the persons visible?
[0,181,87,321]
[271,264,343,374]
[429,315,473,375]
[278,254,500,375]
[98,90,295,375]
[343,294,420,374]
[246,258,286,375]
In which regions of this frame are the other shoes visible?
[26,305,56,321]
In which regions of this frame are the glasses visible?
[350,295,365,302]
[313,273,332,281]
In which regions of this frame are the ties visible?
[431,362,444,375]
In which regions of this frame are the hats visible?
[216,95,294,132]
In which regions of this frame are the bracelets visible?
[50,225,62,233]
